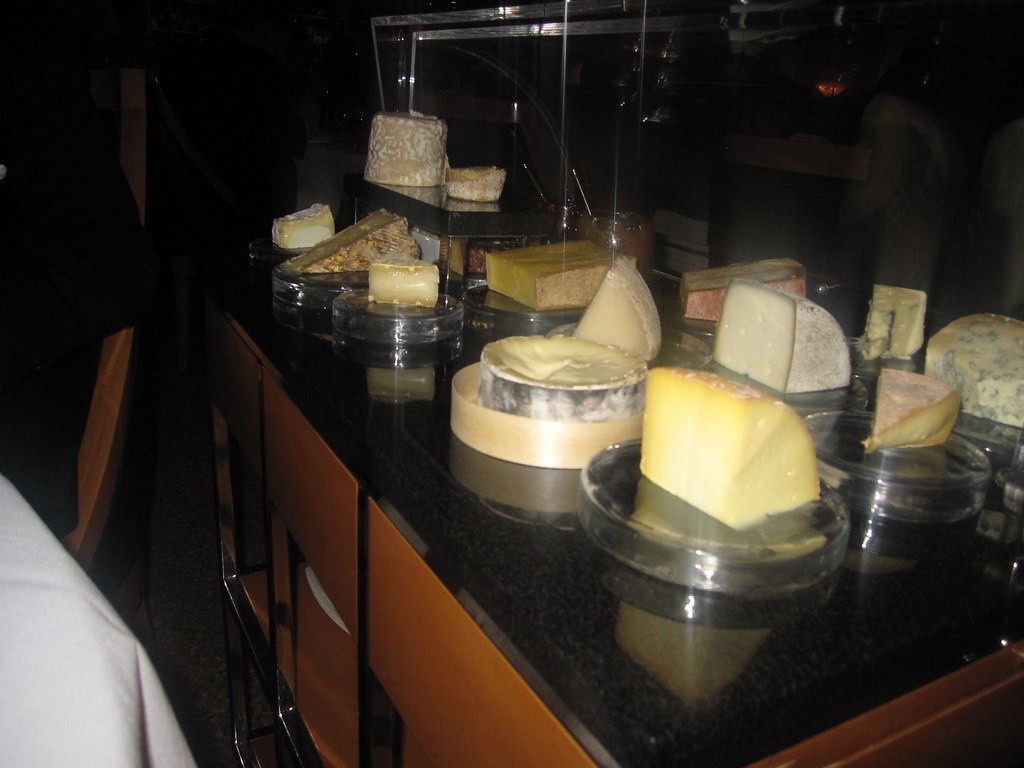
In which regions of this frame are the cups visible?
[578,208,656,301]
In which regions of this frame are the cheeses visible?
[270,114,1024,530]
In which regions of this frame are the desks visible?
[203,296,1024,768]
